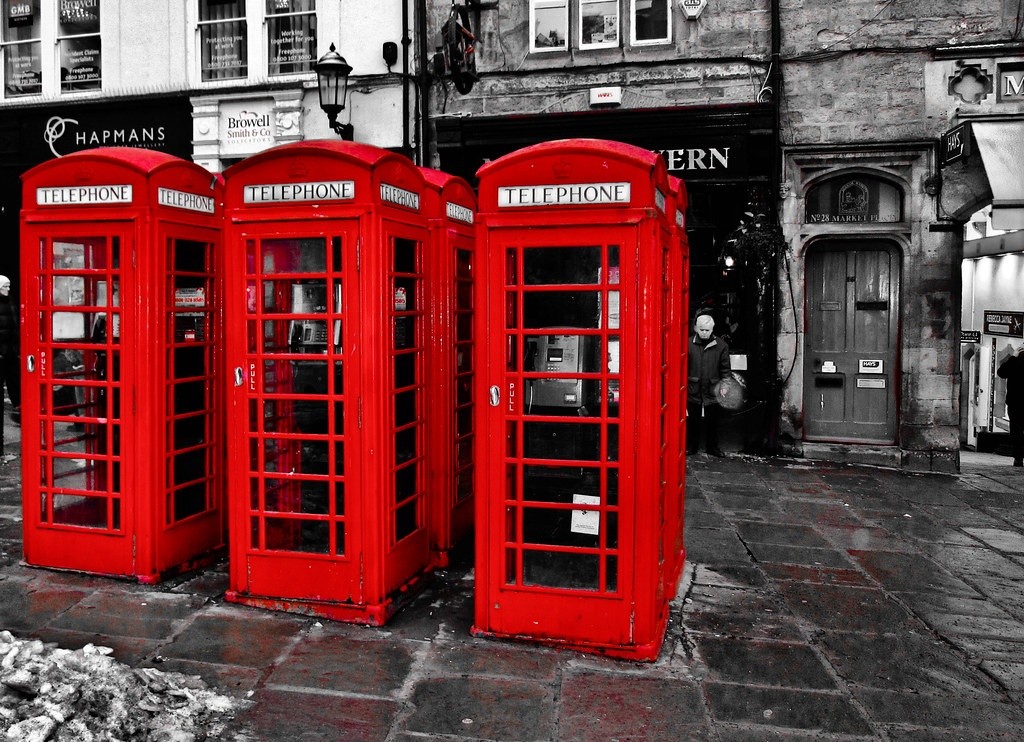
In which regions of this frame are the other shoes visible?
[707,449,725,458]
[686,449,698,456]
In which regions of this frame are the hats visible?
[0,275,10,289]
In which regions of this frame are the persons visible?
[0,276,94,429]
[997,346,1024,467]
[687,315,731,457]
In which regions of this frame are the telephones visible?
[287,283,343,367]
[513,326,595,408]
[90,279,120,352]
